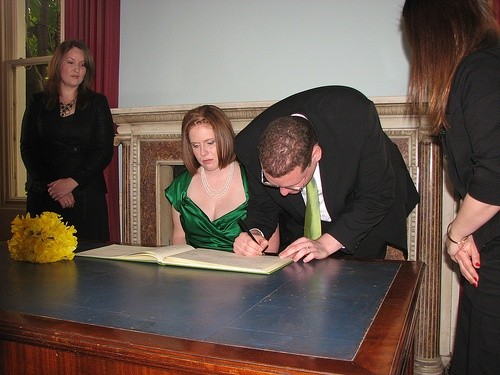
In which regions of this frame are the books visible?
[74,243,295,275]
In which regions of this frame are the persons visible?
[165,105,280,254]
[233,85,420,263]
[401,0,500,375]
[20,40,114,241]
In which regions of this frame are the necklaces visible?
[200,162,235,198]
[59,100,76,117]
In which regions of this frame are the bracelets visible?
[447,222,468,244]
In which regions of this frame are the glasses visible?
[261,164,310,191]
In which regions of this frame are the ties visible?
[304,177,321,241]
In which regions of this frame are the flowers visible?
[6,211,79,265]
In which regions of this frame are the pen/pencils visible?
[236,217,262,247]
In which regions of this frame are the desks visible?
[0,240,426,375]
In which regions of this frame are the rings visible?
[56,193,58,197]
[308,247,311,254]
